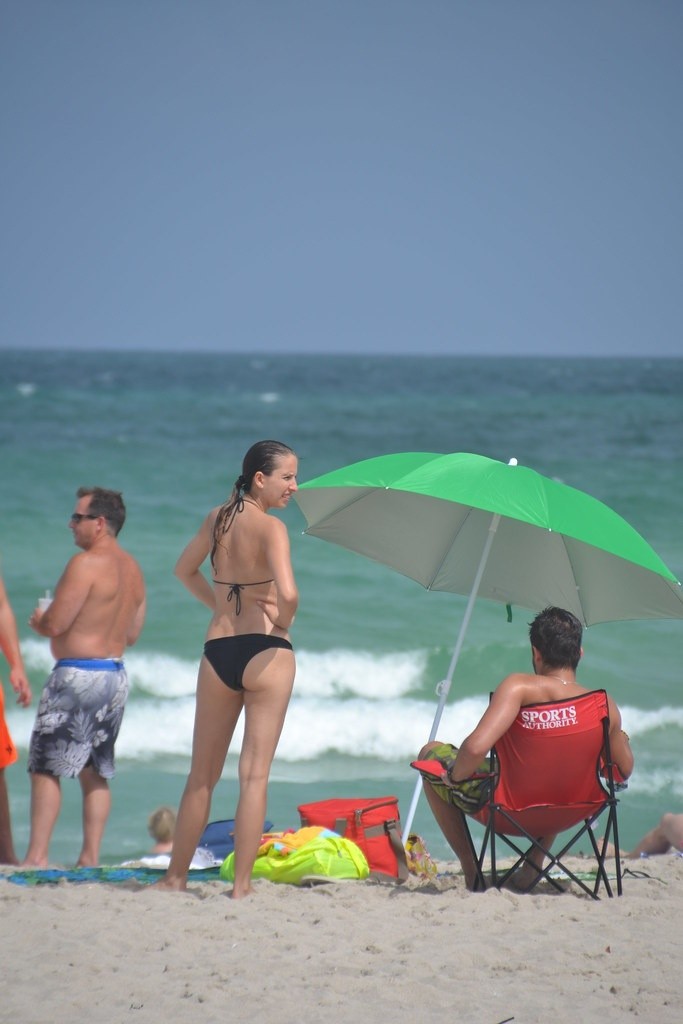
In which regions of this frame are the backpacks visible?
[219,824,372,884]
[406,834,438,885]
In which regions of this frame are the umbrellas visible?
[294,451,683,844]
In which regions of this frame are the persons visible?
[148,806,177,853]
[418,606,633,893]
[134,440,299,899]
[0,575,31,866]
[599,812,683,860]
[20,487,147,870]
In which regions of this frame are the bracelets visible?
[621,730,629,740]
[447,767,469,785]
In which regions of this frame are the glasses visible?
[71,512,98,525]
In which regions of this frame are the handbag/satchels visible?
[297,795,409,884]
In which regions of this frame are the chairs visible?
[409,688,624,903]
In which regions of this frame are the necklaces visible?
[552,676,576,686]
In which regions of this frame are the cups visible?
[38,599,53,614]
